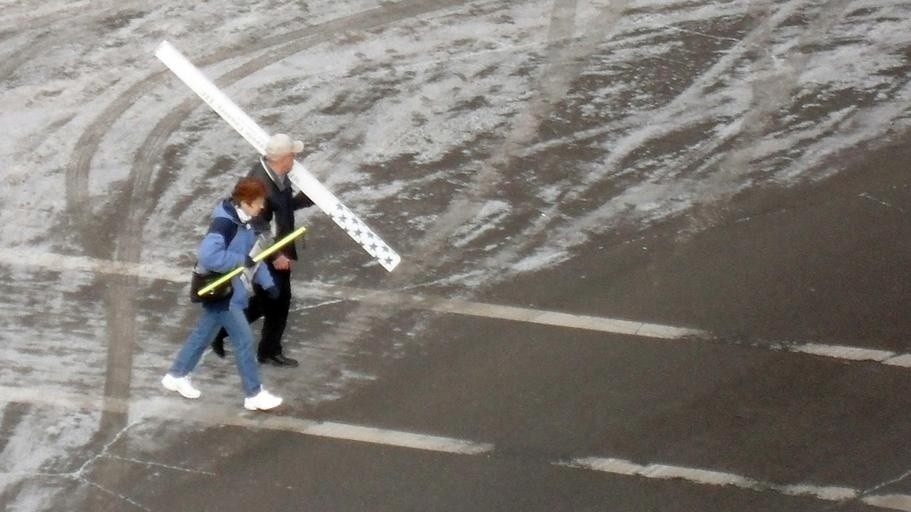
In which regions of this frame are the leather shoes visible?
[258,354,298,368]
[210,338,225,358]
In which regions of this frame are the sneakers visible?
[162,373,201,399]
[243,390,283,411]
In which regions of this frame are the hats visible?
[267,133,305,156]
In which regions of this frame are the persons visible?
[210,134,316,368]
[161,177,285,411]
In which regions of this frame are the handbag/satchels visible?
[192,271,233,302]
